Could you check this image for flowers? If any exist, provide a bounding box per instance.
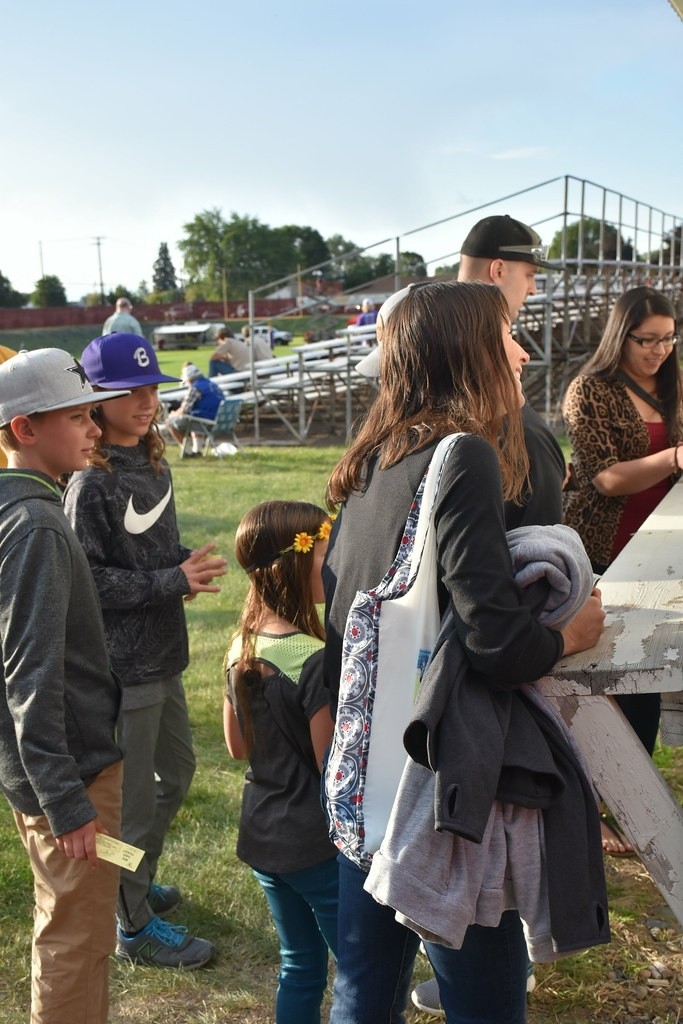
[291,514,339,554]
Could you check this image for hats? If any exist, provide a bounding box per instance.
[0,347,133,428]
[461,215,568,271]
[179,365,202,385]
[80,330,183,389]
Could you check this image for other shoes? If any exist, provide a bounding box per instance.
[411,973,535,1016]
[193,451,204,458]
[176,451,196,458]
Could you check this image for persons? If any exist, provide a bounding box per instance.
[456,216,559,320]
[62,331,229,969]
[101,297,146,340]
[560,286,683,859]
[0,347,128,1024]
[166,365,225,457]
[218,501,410,1024]
[239,327,272,379]
[209,329,253,394]
[312,279,606,1022]
[353,284,566,531]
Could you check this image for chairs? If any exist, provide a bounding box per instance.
[179,398,242,460]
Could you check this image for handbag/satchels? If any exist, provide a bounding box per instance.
[322,433,478,876]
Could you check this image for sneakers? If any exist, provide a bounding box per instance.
[115,882,181,922]
[115,915,214,970]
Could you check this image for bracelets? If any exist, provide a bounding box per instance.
[674,441,683,471]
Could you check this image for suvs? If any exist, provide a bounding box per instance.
[253,326,292,345]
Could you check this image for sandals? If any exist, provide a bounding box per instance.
[597,812,636,857]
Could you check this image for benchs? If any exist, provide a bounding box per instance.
[151,277,683,431]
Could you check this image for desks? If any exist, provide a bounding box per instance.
[527,479,683,933]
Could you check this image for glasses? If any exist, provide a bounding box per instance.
[498,244,549,262]
[627,331,680,348]
